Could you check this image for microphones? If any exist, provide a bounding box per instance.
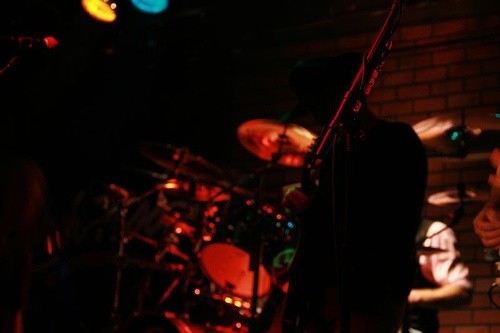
[0,35,60,51]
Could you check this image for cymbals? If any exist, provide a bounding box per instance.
[144,141,232,182]
[426,186,491,207]
[154,176,231,203]
[410,115,500,144]
[235,118,322,168]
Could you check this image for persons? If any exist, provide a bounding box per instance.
[277,55,426,332]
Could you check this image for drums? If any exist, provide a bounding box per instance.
[183,240,270,321]
